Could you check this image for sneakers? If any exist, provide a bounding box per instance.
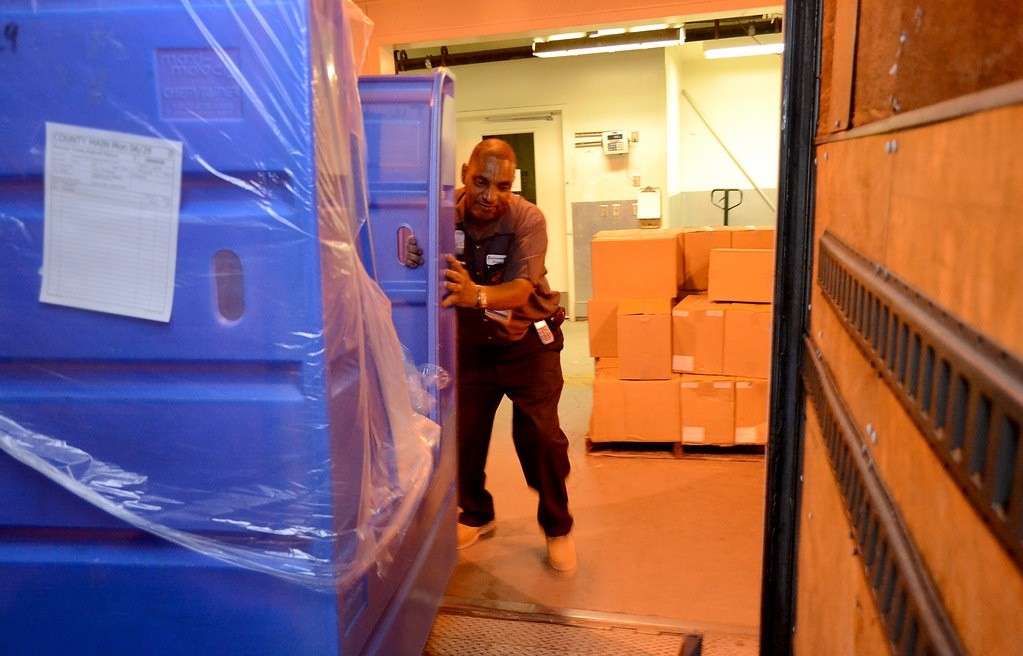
[547,532,577,571]
[456,517,497,550]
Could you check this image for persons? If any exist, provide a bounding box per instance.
[405,139,577,573]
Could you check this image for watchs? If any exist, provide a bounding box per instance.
[476,284,489,311]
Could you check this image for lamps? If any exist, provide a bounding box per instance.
[702,34,784,61]
[530,23,686,57]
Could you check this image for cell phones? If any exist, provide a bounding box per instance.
[534,320,555,344]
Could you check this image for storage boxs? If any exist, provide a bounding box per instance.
[588,234,679,299]
[589,299,617,357]
[735,377,770,443]
[614,298,673,380]
[707,248,775,304]
[626,373,681,442]
[685,225,732,291]
[681,373,735,444]
[594,369,626,443]
[723,302,773,379]
[595,356,618,369]
[591,230,685,290]
[670,293,732,375]
[733,225,775,249]
